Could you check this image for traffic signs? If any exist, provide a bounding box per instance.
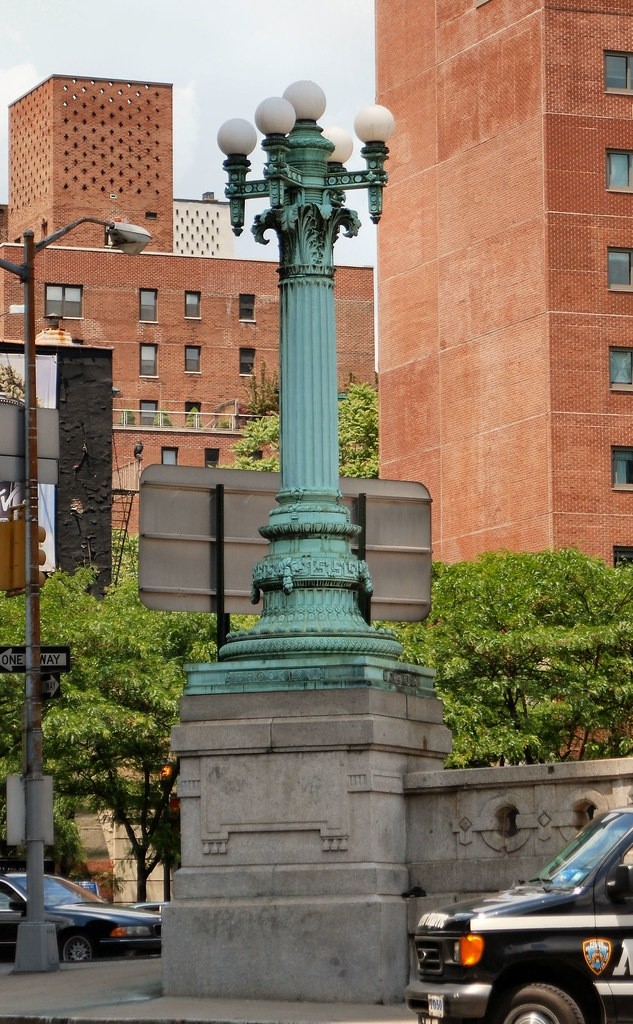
[40,673,62,700]
[0,645,71,674]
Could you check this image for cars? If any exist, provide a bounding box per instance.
[0,872,161,961]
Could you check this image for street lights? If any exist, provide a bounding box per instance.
[23,519,47,590]
[0,215,154,976]
[186,77,442,694]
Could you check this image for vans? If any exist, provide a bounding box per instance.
[403,804,633,1024]
